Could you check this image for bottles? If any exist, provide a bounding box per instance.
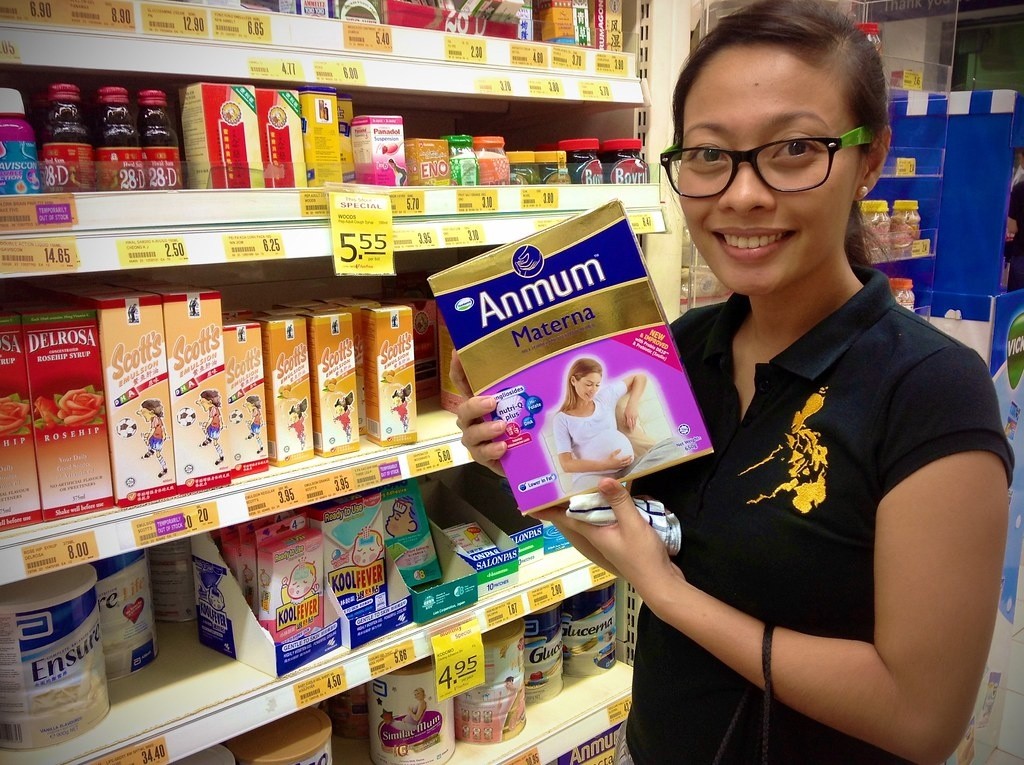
[137,90,183,192]
[0,82,95,195]
[859,200,891,255]
[95,87,145,194]
[891,200,920,255]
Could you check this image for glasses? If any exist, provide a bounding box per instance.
[660,126,872,198]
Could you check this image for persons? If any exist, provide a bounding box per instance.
[497,676,519,737]
[553,354,645,496]
[389,159,407,186]
[1008,148,1024,291]
[449,1,1017,764]
[1004,160,1024,271]
[390,687,427,732]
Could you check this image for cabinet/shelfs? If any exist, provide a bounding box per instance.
[0,0,691,765]
[681,0,958,324]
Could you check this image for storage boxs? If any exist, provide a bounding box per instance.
[0,276,475,530]
[191,467,572,680]
[240,0,623,49]
[427,201,715,517]
[178,81,407,188]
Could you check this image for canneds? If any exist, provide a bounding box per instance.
[170,579,618,765]
[443,134,652,184]
[0,537,197,752]
[861,200,921,313]
